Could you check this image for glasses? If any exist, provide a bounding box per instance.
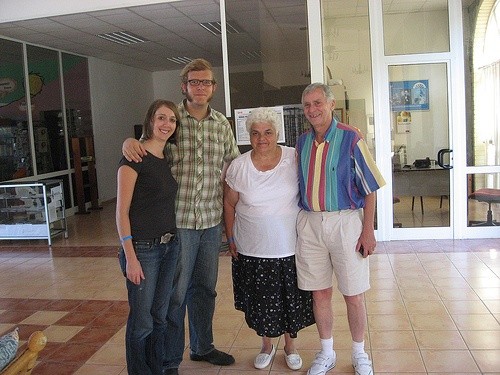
[187,79,214,86]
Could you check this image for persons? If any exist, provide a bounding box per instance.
[122,59,241,375]
[294,82,386,375]
[224,108,316,370]
[115,100,181,375]
[402,90,412,104]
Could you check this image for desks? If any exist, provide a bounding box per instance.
[393,165,449,215]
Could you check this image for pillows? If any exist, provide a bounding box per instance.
[0,327,19,371]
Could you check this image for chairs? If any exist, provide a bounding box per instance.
[0,330,47,375]
[468,188,500,226]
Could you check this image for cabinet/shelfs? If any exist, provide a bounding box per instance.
[0,179,69,246]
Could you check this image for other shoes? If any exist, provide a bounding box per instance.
[284,346,302,370]
[254,344,275,369]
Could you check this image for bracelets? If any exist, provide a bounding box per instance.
[228,238,233,243]
[121,235,132,241]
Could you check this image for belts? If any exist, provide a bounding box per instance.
[133,232,175,244]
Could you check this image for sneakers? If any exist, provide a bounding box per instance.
[190,349,235,366]
[351,353,373,375]
[306,350,336,375]
